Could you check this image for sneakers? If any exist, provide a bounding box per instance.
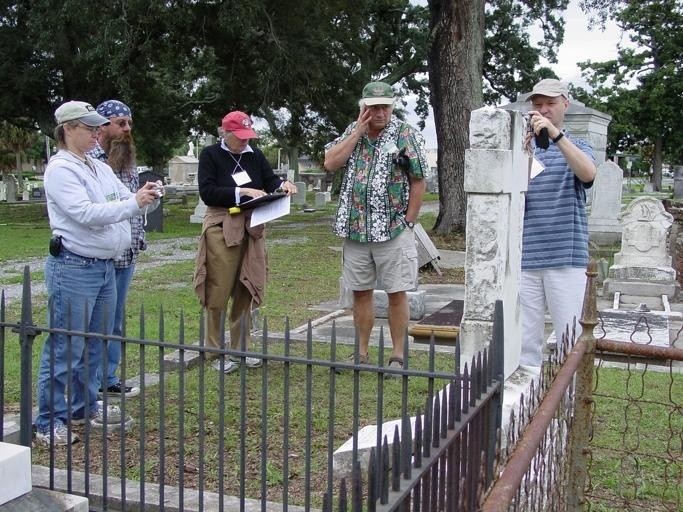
[98,383,139,397]
[384,357,403,379]
[35,423,80,446]
[229,355,263,368]
[334,352,368,374]
[70,399,103,426]
[205,359,237,374]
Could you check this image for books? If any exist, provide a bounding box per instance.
[236,191,291,229]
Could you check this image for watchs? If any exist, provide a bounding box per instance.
[405,220,415,228]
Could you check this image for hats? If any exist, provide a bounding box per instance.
[53,100,110,127]
[222,111,257,139]
[525,78,568,101]
[362,82,393,106]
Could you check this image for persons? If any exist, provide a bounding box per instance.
[517,75,599,422]
[33,98,165,447]
[195,110,301,373]
[85,99,145,398]
[324,79,423,383]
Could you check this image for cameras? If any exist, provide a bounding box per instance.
[522,113,534,132]
[151,183,164,199]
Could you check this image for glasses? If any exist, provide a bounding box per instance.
[77,127,99,132]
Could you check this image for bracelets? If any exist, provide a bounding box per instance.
[550,128,567,144]
